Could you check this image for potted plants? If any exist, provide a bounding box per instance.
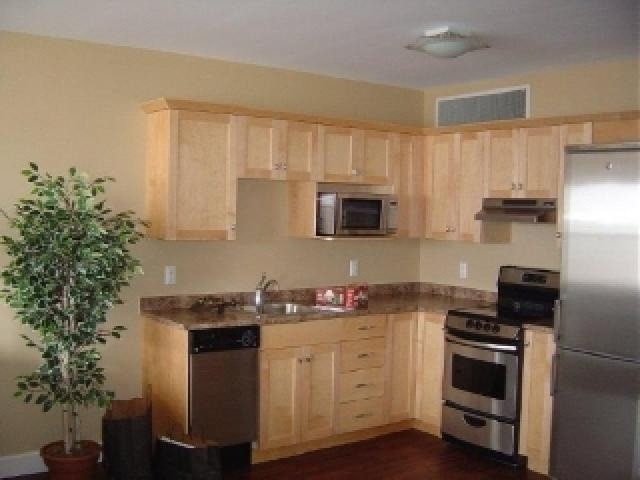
[0,160,148,480]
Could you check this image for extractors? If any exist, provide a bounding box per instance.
[473,197,557,224]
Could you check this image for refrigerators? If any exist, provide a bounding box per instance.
[547,140,640,480]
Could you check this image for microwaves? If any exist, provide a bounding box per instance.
[320,192,400,235]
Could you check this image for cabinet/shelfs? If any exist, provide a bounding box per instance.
[488,117,560,199]
[237,106,317,180]
[338,313,390,445]
[390,309,419,433]
[426,124,512,243]
[317,114,399,185]
[522,323,555,476]
[251,319,338,464]
[142,98,237,241]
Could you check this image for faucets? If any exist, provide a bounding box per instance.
[255,276,282,307]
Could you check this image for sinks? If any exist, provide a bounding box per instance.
[244,302,327,315]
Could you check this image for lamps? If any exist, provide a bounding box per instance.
[405,32,491,58]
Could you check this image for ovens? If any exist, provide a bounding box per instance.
[496,266,560,328]
[442,328,519,425]
